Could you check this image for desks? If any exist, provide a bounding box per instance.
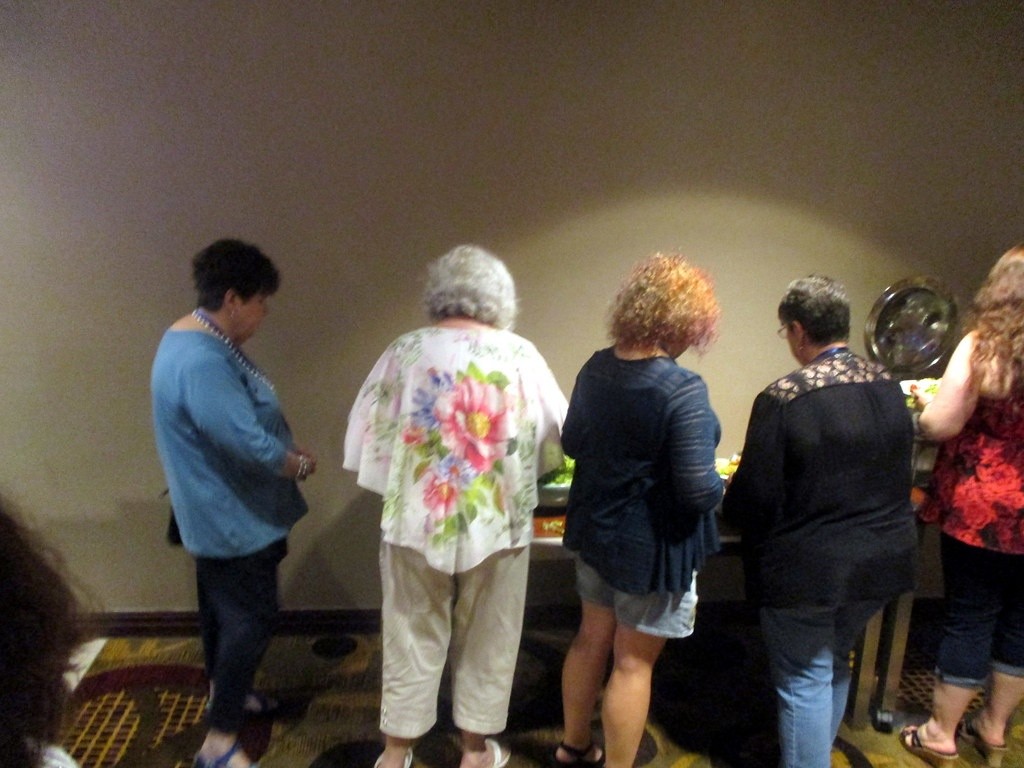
[528,482,942,732]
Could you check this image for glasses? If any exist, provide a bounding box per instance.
[778,319,804,339]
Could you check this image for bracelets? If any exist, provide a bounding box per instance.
[296,454,307,479]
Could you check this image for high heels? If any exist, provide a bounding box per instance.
[957,717,1009,768]
[899,723,959,768]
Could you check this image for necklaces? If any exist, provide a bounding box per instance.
[190,307,278,397]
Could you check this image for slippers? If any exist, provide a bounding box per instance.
[374,745,413,768]
[459,738,512,768]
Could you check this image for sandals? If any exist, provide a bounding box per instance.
[206,692,280,712]
[193,741,257,768]
[554,739,606,768]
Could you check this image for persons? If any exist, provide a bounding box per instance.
[725,275,914,768]
[149,237,318,767]
[0,498,106,768]
[900,246,1024,766]
[559,254,725,768]
[352,244,561,768]
